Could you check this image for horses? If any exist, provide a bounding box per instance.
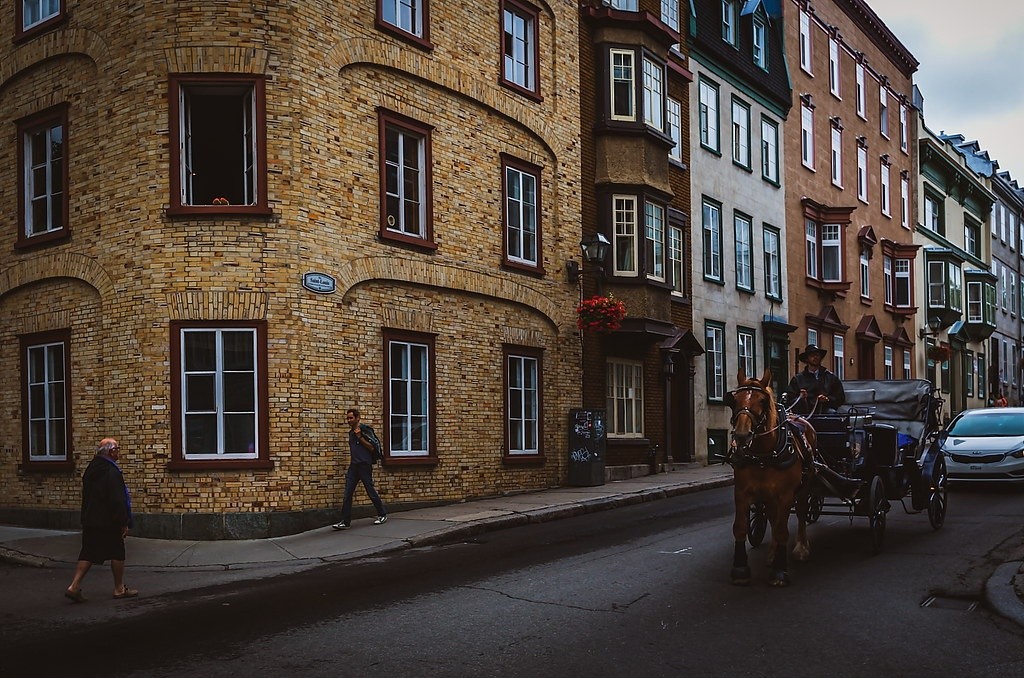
[730,367,817,587]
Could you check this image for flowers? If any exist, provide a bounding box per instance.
[928,346,954,363]
[577,292,626,338]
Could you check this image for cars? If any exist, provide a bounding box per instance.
[940,407,1024,486]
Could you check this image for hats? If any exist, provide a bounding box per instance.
[799,344,828,363]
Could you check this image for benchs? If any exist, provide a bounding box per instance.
[825,379,932,461]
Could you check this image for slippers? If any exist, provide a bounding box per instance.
[65,589,88,603]
[113,584,139,598]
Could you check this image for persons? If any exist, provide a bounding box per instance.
[988,392,1007,407]
[64,438,138,601]
[333,409,387,529]
[789,344,846,415]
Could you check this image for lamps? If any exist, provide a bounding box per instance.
[661,354,674,381]
[566,233,610,283]
[920,315,942,339]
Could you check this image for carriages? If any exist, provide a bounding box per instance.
[727,378,948,583]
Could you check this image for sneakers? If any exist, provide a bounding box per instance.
[374,516,388,525]
[332,520,354,529]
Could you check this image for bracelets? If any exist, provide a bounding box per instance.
[358,434,363,441]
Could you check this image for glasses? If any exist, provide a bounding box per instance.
[112,447,120,452]
